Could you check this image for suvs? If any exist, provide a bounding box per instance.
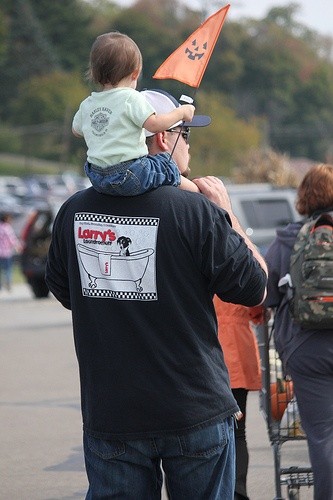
[229,184,306,256]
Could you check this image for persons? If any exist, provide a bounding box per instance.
[44,88,269,500]
[0,212,23,291]
[71,32,202,194]
[210,293,263,500]
[264,165,333,500]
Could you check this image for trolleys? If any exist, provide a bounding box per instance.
[255,307,314,500]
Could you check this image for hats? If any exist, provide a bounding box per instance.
[137,87,212,137]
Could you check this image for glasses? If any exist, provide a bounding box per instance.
[168,126,191,143]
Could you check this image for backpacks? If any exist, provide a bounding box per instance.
[278,212,333,331]
